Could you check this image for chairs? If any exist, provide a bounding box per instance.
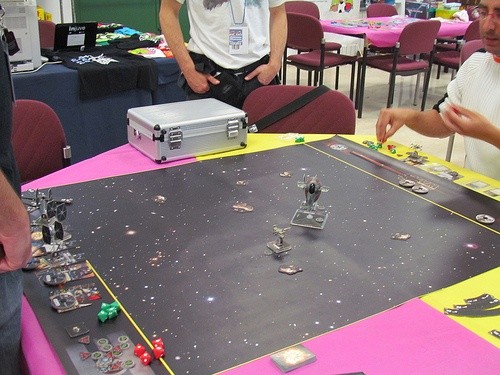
[243,0,500,134]
[12,99,71,186]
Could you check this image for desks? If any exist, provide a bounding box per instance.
[20,133,500,375]
[320,15,473,118]
[11,24,188,165]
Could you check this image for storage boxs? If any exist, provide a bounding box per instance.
[127,98,248,164]
[435,8,458,18]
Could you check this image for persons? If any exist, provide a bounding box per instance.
[376,0,500,181]
[158,0,288,109]
[0,37,32,375]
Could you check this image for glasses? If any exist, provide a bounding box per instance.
[468,6,500,20]
